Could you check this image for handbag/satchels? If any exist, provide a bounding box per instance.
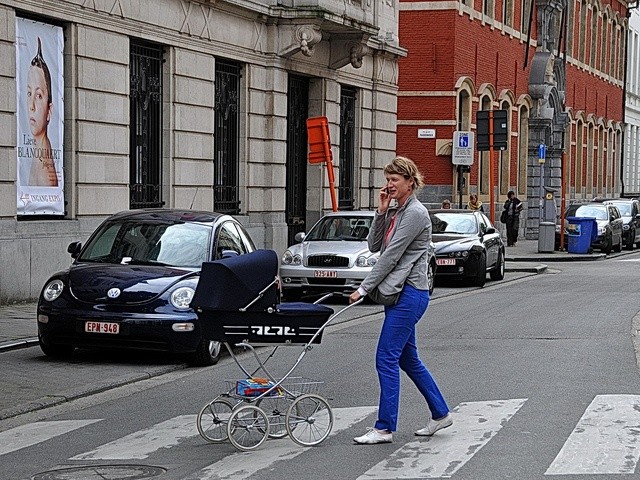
[368,287,400,306]
[500,208,509,223]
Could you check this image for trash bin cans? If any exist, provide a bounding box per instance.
[538,222,556,253]
[566,217,598,253]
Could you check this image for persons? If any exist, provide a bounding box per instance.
[464,194,484,214]
[27,36,58,185]
[349,157,453,445]
[441,200,451,210]
[504,191,524,248]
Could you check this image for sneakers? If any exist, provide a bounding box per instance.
[353,426,393,444]
[414,414,453,436]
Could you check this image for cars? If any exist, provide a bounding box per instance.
[280,209,437,304]
[592,196,640,251]
[423,209,507,289]
[553,202,623,253]
[36,208,257,367]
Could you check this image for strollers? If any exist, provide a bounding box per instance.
[185,249,366,451]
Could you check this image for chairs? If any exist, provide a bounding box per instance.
[350,224,370,239]
[333,226,353,239]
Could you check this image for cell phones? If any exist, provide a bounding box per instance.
[385,187,389,195]
